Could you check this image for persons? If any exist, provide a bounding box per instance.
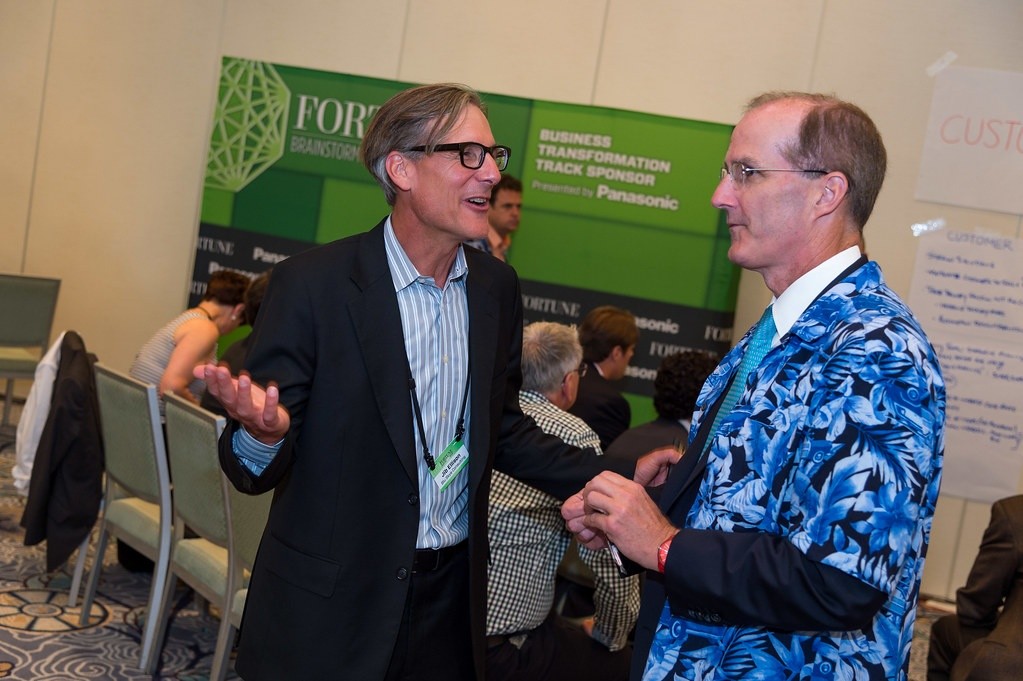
[486,306,720,681]
[561,91,946,681]
[193,83,683,681]
[128,269,272,425]
[927,490,1022,681]
[462,173,524,265]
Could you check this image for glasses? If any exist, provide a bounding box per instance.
[408,141,511,172]
[720,161,830,189]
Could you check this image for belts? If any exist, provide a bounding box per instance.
[410,540,468,580]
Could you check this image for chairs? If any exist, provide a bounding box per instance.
[57,333,176,675]
[141,389,253,681]
[208,427,276,681]
[0,274,60,427]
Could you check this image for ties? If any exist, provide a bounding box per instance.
[699,304,778,458]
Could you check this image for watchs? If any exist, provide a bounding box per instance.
[658,528,682,574]
